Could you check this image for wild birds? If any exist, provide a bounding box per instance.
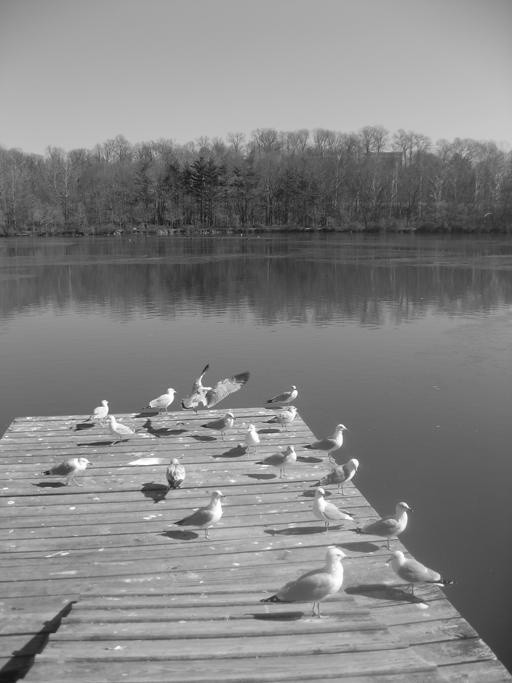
[309,458,359,496]
[301,423,349,465]
[86,387,177,447]
[167,458,185,489]
[312,487,360,534]
[180,364,298,479]
[386,551,456,597]
[41,457,93,487]
[348,502,413,551]
[259,547,351,619]
[173,490,226,541]
